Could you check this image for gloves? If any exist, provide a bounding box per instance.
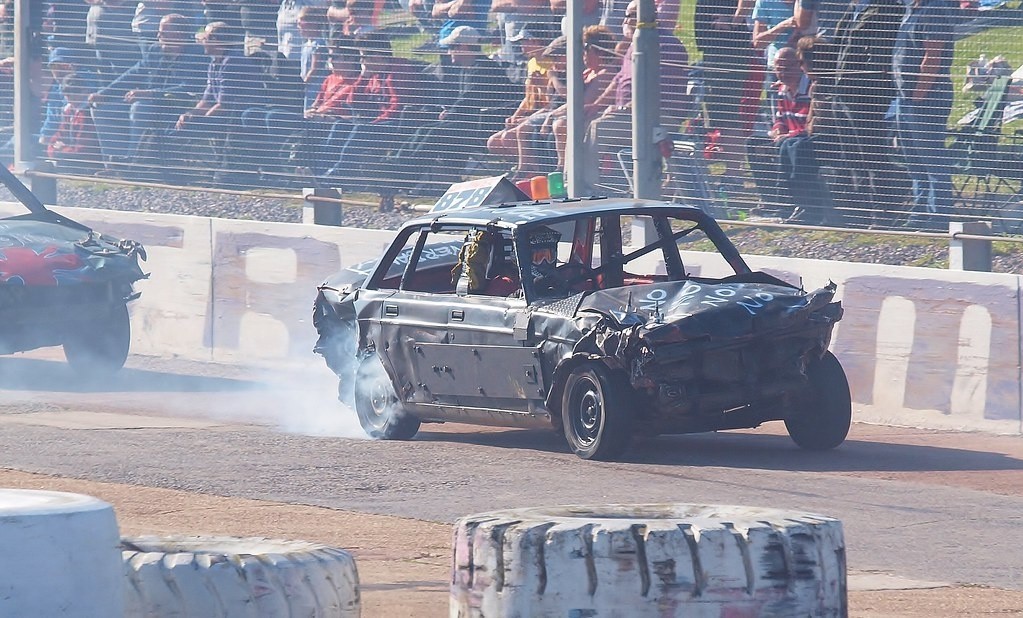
[533,278,546,295]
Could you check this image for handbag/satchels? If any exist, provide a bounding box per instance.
[963,60,1013,90]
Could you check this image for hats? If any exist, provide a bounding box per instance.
[440,26,479,46]
[47,46,73,67]
[510,28,534,41]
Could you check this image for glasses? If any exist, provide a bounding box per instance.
[447,45,461,51]
[531,248,558,265]
[63,88,82,94]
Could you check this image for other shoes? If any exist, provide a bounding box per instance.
[902,219,947,229]
[296,166,319,189]
[790,210,819,220]
[95,170,122,177]
[511,175,535,183]
[751,208,766,215]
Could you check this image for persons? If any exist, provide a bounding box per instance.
[486,225,604,297]
[1,0,953,232]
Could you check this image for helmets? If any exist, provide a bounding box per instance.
[510,226,562,278]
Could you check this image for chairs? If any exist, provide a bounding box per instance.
[947,76,1011,233]
[484,232,515,279]
[464,98,520,181]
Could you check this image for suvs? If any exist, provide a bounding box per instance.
[312,195,852,462]
[0,166,153,381]
